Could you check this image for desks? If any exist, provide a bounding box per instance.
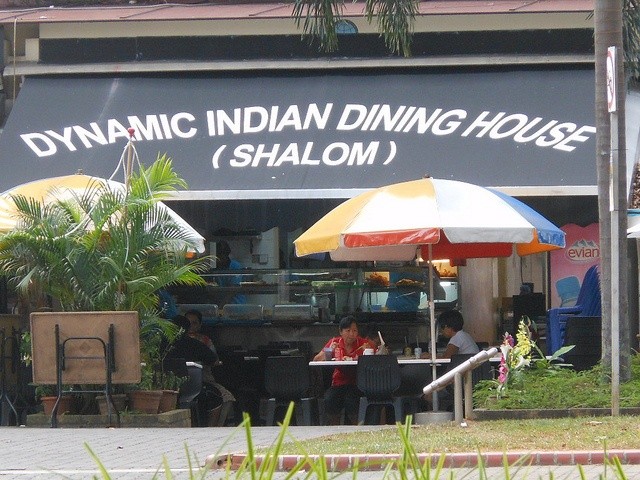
[30,310,141,426]
[1,313,34,424]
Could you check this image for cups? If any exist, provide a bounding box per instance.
[324,348,332,361]
[318,308,331,322]
[404,347,412,357]
[363,349,374,355]
[414,348,422,358]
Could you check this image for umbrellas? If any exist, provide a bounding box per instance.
[1,169,207,258]
[293,172,567,413]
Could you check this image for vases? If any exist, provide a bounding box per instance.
[94,393,128,413]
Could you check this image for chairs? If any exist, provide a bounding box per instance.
[356,356,401,426]
[152,356,204,426]
[546,263,601,357]
[442,353,492,418]
[259,341,312,351]
[264,356,311,425]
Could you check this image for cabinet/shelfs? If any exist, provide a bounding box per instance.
[174,267,431,327]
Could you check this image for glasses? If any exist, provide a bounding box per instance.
[441,324,448,329]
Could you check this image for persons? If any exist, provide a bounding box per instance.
[420,310,480,410]
[385,270,446,311]
[183,309,237,427]
[211,240,248,305]
[313,316,381,425]
[155,315,223,427]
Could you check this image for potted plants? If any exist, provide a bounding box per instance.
[32,383,78,417]
[127,369,162,415]
[159,370,177,415]
